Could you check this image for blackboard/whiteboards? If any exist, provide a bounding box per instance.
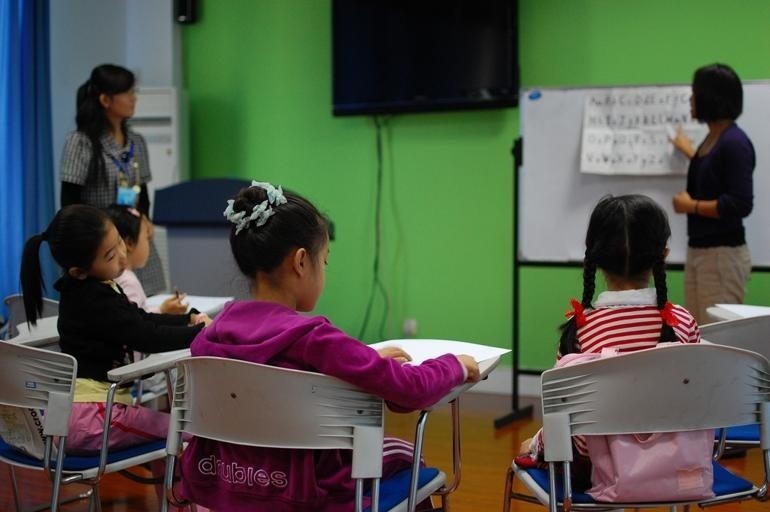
[519,78,770,273]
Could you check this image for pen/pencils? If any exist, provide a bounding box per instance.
[176,290,179,298]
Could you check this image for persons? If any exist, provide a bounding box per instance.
[180,181,480,512]
[666,63,756,327]
[19,206,214,511]
[59,64,167,297]
[519,194,702,481]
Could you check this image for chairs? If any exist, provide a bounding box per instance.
[503,303,769,512]
[0,294,238,512]
[161,337,501,512]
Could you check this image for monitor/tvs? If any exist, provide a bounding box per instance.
[331,0,520,117]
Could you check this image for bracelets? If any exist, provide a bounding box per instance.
[695,199,699,214]
[195,312,208,325]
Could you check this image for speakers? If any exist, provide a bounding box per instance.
[171,0,203,25]
